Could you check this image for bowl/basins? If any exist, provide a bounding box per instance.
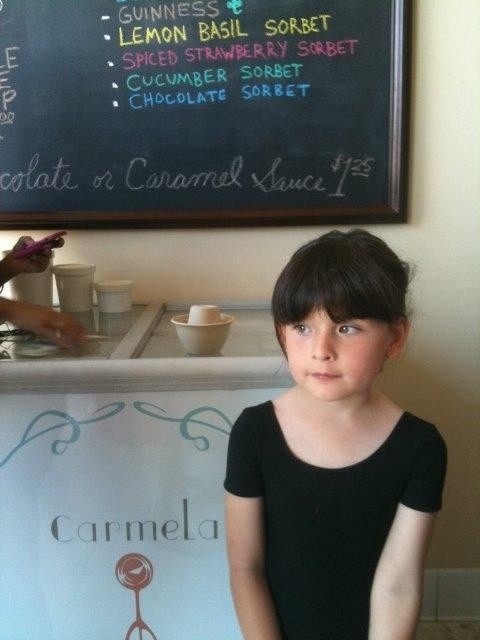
[169,313,235,355]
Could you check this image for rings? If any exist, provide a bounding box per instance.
[56,329,62,338]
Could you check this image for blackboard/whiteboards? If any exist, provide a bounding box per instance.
[0,0,413,231]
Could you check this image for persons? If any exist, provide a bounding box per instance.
[221,227,448,640]
[0,235,85,351]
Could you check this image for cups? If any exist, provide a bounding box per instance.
[51,262,97,314]
[94,279,135,313]
[3,249,55,311]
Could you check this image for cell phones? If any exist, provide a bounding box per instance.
[13,229,66,260]
